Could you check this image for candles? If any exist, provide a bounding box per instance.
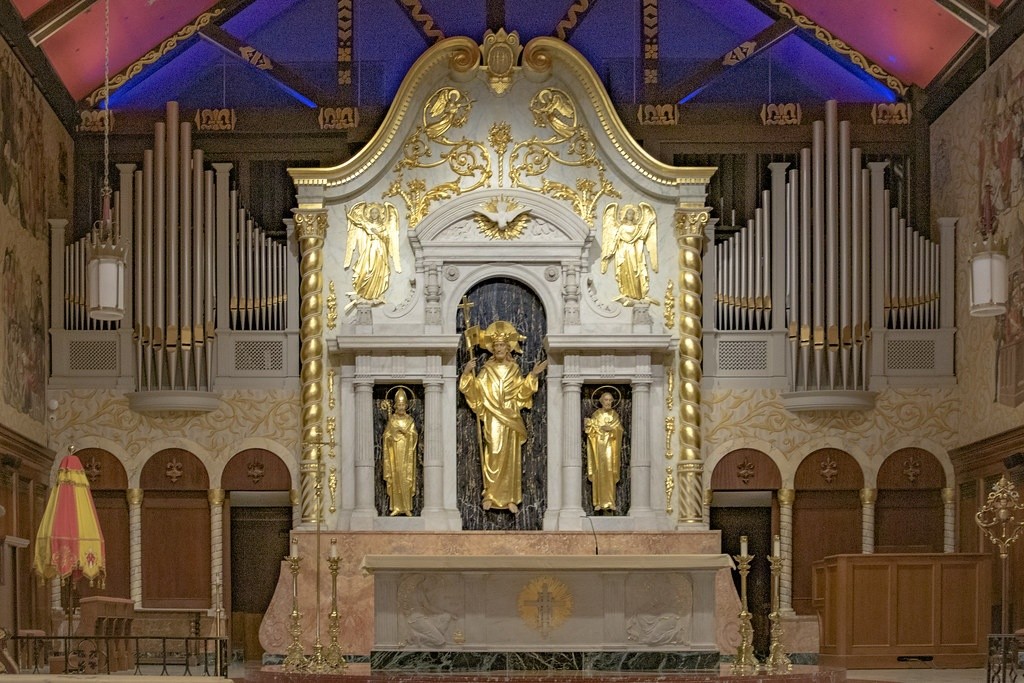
[773,535,780,556]
[330,537,338,558]
[292,536,298,557]
[216,572,219,584]
[739,536,748,558]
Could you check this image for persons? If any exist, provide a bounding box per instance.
[533,92,578,140]
[614,208,649,301]
[426,90,478,136]
[458,321,549,514]
[381,384,419,518]
[352,208,390,301]
[583,392,624,511]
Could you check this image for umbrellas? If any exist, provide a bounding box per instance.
[28,442,109,658]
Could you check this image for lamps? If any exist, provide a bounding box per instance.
[969,0,1009,318]
[85,0,125,319]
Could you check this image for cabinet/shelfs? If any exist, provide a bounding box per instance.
[812,553,994,670]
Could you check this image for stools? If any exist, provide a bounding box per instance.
[17,629,45,669]
